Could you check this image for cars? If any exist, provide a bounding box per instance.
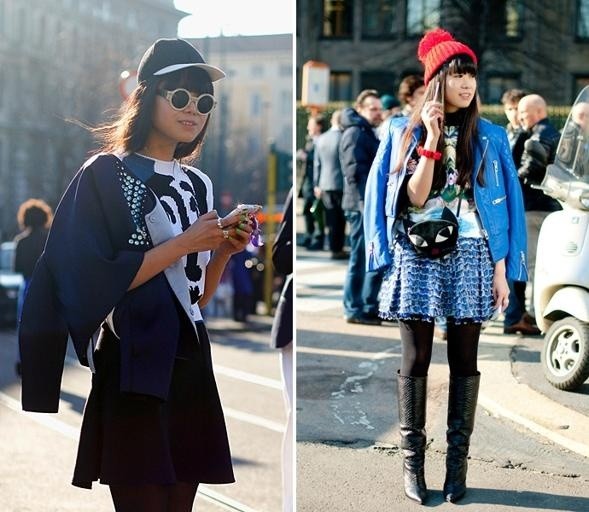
[245,201,286,314]
[0,240,27,328]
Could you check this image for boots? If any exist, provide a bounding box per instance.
[443,371,480,504]
[397,371,429,505]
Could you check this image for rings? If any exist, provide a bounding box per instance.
[216,218,222,229]
[222,231,229,239]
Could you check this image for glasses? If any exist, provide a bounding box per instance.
[157,84,218,116]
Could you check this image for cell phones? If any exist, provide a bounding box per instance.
[223,203,262,230]
[434,82,443,129]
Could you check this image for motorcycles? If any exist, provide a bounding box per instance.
[522,82,589,395]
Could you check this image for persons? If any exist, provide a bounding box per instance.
[361,29,530,504]
[14,200,54,379]
[228,241,255,323]
[17,37,261,512]
[296,74,560,336]
[558,102,588,182]
[270,181,294,509]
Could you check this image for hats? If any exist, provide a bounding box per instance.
[137,38,226,87]
[417,28,479,86]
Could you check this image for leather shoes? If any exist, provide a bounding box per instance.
[504,314,542,336]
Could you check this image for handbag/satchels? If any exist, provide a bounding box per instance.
[401,206,459,261]
